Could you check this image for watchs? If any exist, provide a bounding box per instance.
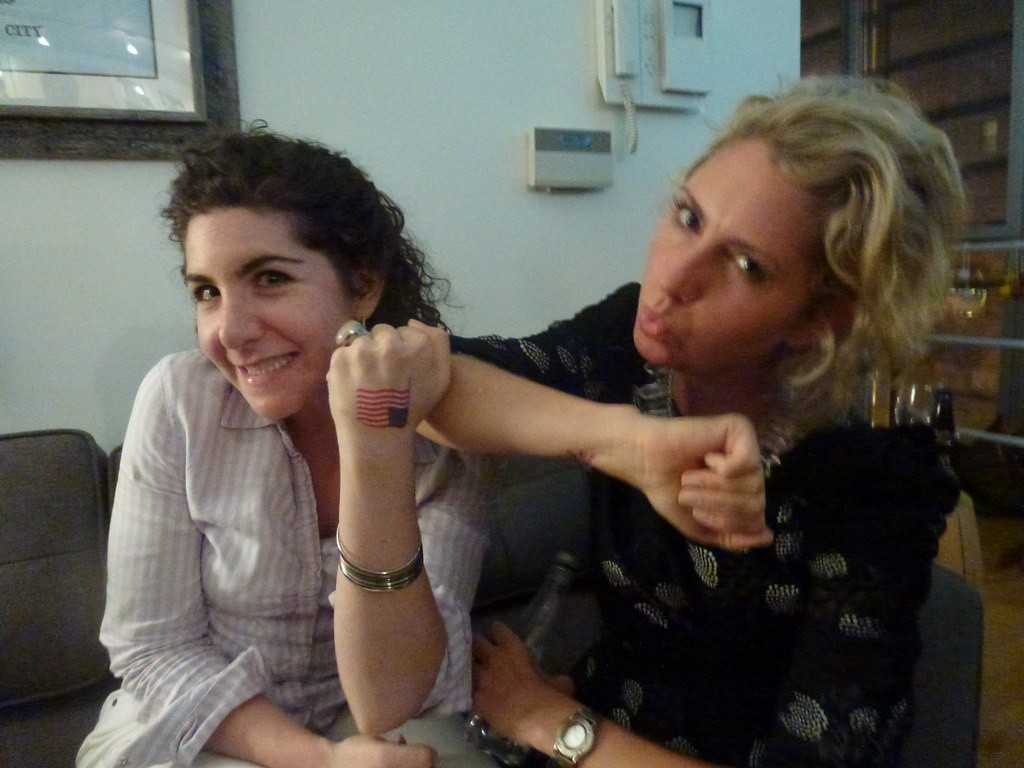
[550,708,606,768]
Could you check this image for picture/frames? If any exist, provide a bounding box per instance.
[0,0,241,162]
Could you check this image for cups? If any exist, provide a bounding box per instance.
[894,383,931,427]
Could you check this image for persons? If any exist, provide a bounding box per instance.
[72,123,506,768]
[409,73,965,768]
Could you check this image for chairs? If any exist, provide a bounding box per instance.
[0,427,124,768]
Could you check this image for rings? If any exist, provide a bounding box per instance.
[337,325,372,347]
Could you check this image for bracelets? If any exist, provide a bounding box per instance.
[335,520,425,593]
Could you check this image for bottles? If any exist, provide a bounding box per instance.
[931,389,967,491]
[465,552,579,763]
